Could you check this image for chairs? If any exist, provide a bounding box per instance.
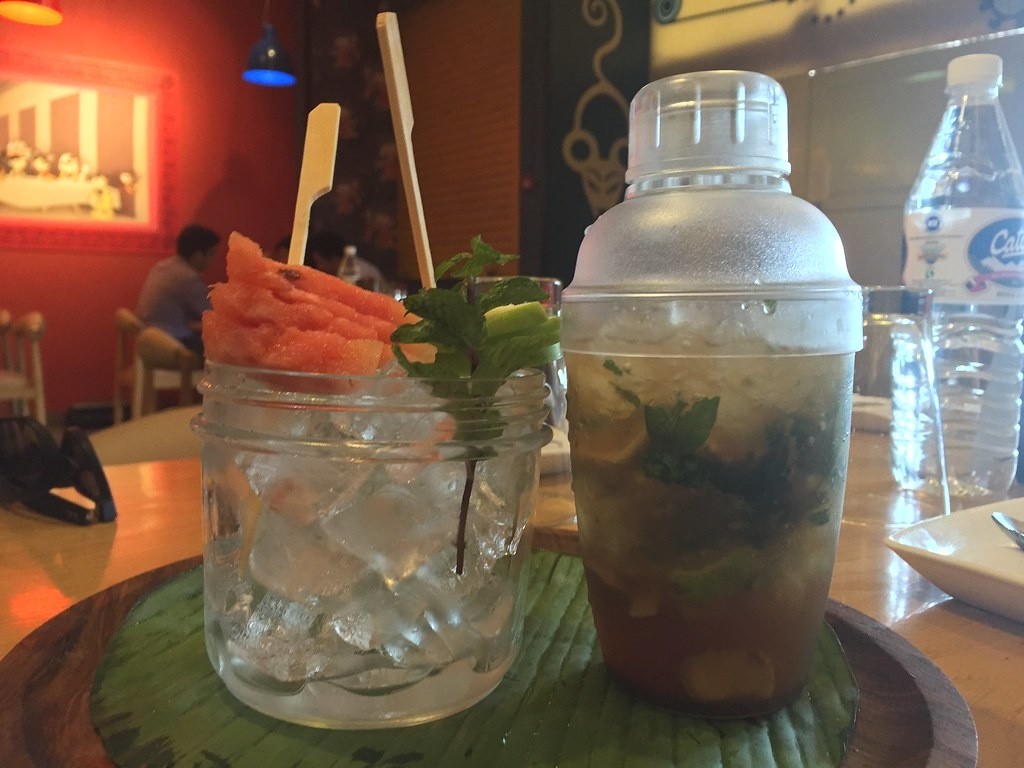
[112,306,200,426]
[0,307,49,427]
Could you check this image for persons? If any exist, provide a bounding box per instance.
[135,224,222,352]
[272,171,386,295]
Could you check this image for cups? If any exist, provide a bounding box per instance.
[191,360,553,730]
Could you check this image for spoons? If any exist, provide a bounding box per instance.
[990,511,1024,549]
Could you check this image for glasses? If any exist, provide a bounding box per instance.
[1,416,118,526]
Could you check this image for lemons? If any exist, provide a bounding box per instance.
[575,408,650,472]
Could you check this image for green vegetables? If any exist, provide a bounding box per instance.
[388,238,838,577]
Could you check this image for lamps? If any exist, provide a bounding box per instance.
[241,0,297,87]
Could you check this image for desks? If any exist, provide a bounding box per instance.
[0,426,1023,768]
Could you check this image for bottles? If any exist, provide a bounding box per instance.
[889,52,1023,498]
[558,70,863,721]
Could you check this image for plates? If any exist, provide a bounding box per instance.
[885,499,1023,627]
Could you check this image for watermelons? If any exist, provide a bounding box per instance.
[199,231,429,393]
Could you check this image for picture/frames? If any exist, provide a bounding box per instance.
[0,47,176,257]
